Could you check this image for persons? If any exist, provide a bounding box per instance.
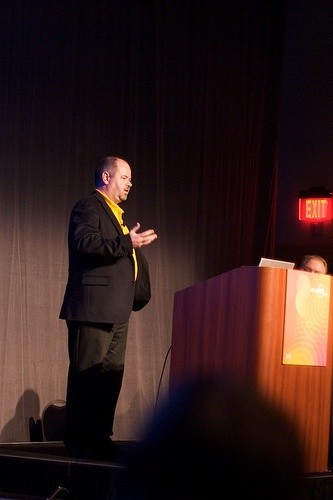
[300,255,327,275]
[59,158,157,460]
